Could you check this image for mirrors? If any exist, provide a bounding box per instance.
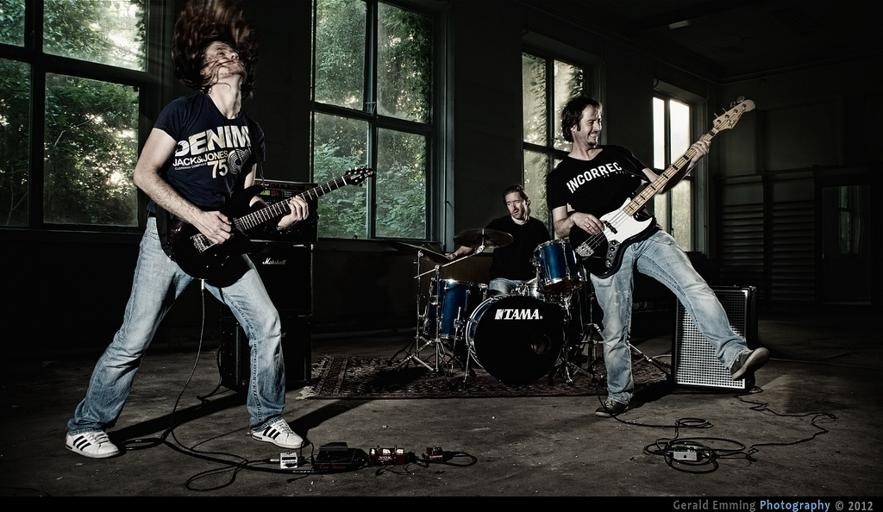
[815,175,878,314]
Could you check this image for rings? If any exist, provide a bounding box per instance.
[585,224,591,228]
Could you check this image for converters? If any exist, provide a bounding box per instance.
[314,447,360,471]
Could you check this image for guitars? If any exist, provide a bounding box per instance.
[569,96,757,279]
[169,166,377,280]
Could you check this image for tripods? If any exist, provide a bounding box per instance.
[549,287,672,385]
[389,250,485,383]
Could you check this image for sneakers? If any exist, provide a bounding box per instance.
[596,401,627,419]
[730,348,769,381]
[65,430,119,459]
[252,417,304,450]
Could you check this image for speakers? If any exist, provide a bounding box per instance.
[220,239,315,394]
[672,285,758,392]
[253,178,319,241]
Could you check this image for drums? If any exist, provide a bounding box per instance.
[522,281,571,320]
[423,278,482,341]
[531,241,587,294]
[466,294,568,389]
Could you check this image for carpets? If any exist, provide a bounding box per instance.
[296,350,671,402]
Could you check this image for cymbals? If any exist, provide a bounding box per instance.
[400,241,451,265]
[454,227,513,249]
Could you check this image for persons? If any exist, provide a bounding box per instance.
[481,185,553,296]
[549,97,772,419]
[65,1,317,460]
